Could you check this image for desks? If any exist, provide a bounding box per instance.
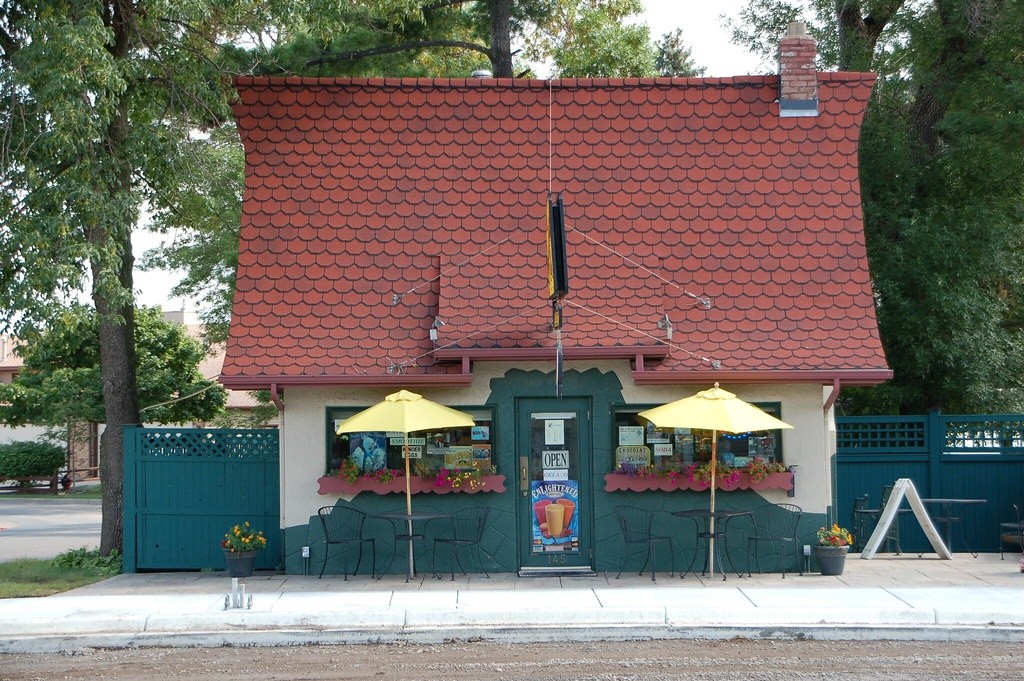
[855,508,913,550]
[671,509,754,581]
[920,498,988,558]
[371,511,449,580]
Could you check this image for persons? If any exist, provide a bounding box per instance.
[351,435,386,472]
[435,434,447,448]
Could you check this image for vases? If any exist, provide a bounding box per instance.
[225,551,257,577]
[815,546,850,575]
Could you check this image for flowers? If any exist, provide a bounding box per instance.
[221,522,267,553]
[819,523,853,548]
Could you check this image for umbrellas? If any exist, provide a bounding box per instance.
[637,381,793,583]
[337,389,476,579]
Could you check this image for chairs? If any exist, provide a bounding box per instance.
[614,505,674,581]
[852,485,904,556]
[318,505,375,579]
[745,503,804,578]
[998,504,1024,560]
[432,506,490,580]
